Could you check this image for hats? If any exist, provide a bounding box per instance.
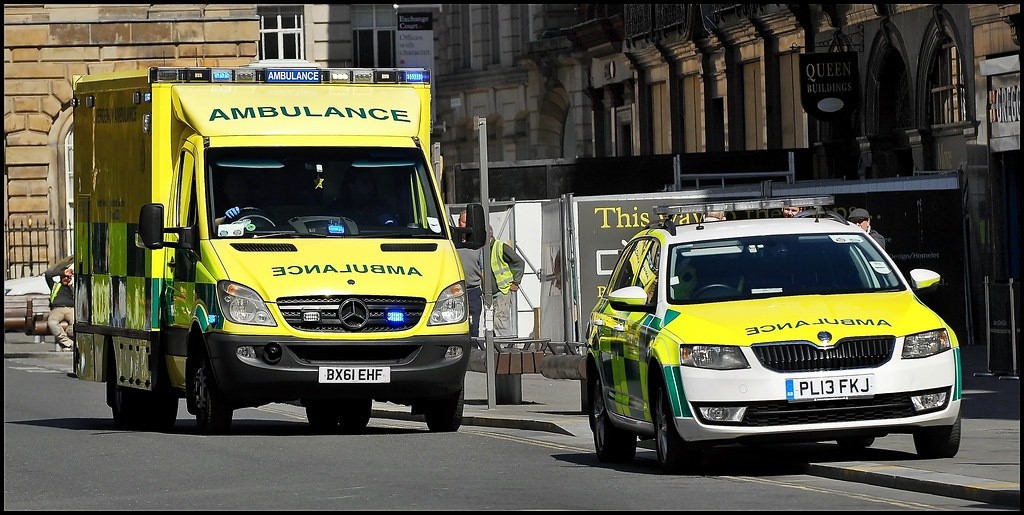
[848,208,872,219]
[490,225,493,237]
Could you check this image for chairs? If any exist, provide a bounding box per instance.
[320,172,397,226]
[781,252,839,290]
[682,257,744,296]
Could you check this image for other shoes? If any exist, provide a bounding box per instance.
[55,341,63,352]
[63,346,73,352]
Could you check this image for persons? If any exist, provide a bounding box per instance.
[702,211,727,222]
[215,174,277,224]
[452,210,483,337]
[45,268,75,353]
[848,209,887,250]
[781,206,802,219]
[321,171,402,227]
[478,225,525,347]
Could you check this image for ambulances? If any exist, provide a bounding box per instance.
[581,194,964,475]
[66,56,491,439]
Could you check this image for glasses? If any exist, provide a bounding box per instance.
[226,182,249,188]
[850,218,868,224]
[61,274,71,279]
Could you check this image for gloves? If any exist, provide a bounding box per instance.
[224,206,240,219]
